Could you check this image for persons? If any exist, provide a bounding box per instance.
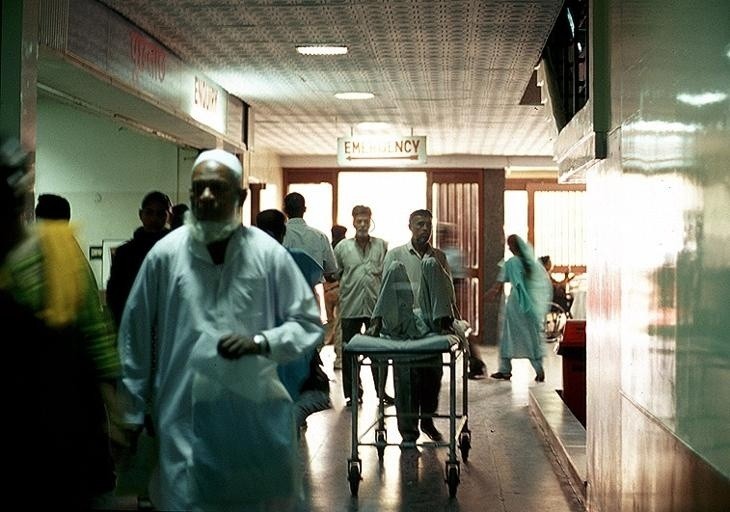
[34,192,71,225]
[282,192,340,283]
[255,209,332,427]
[538,255,577,315]
[483,234,553,382]
[0,122,124,511]
[364,257,487,379]
[334,204,396,406]
[315,224,348,369]
[367,208,465,447]
[435,221,466,322]
[115,148,322,512]
[105,190,172,338]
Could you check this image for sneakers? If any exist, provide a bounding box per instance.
[492,373,511,379]
[401,433,419,449]
[421,419,441,441]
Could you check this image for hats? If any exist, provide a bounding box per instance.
[192,149,242,177]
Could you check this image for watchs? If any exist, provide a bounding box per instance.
[253,333,268,356]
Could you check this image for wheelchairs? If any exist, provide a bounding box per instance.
[536,291,576,343]
